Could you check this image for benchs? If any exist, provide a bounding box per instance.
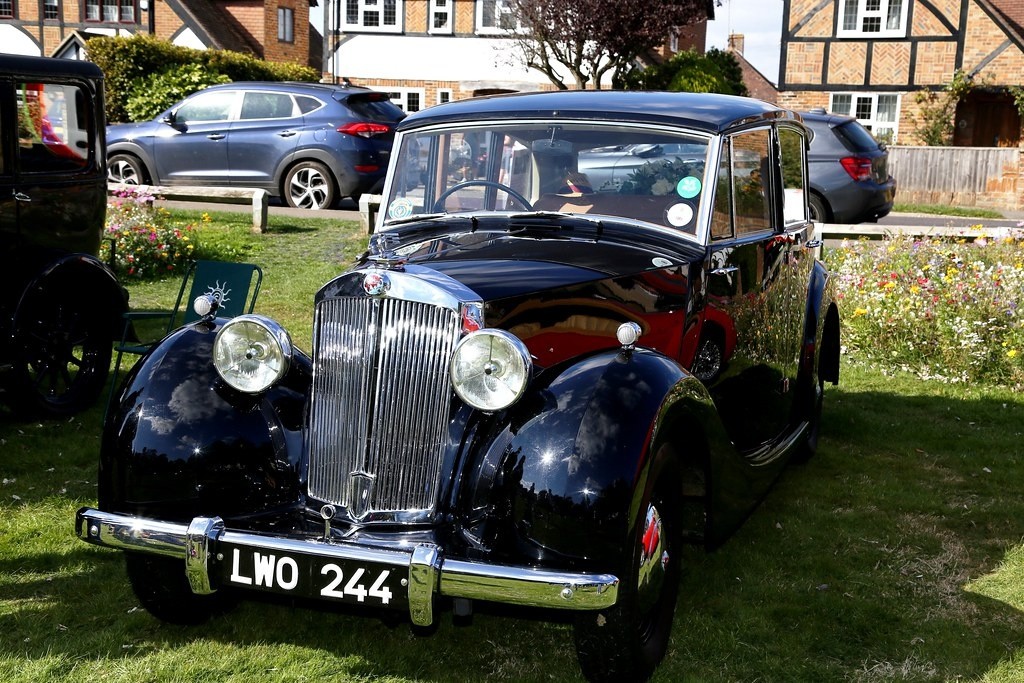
[533,193,717,235]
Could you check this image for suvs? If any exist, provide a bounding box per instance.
[74,91,840,683]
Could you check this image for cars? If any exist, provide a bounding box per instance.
[104,79,418,209]
[578,106,897,224]
[0,52,130,423]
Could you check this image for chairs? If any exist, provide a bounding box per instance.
[103,260,262,424]
